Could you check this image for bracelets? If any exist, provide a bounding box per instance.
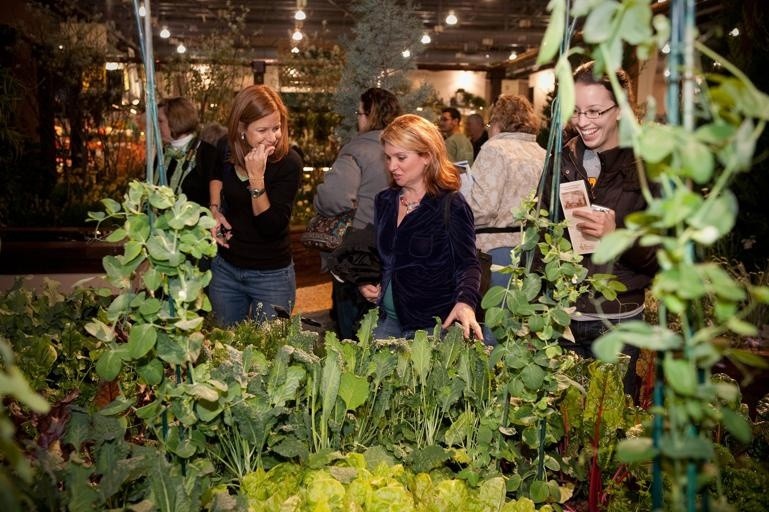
[208,202,220,210]
[246,175,266,181]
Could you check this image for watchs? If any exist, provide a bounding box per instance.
[245,185,268,199]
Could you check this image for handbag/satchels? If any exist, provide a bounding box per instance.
[298,153,364,252]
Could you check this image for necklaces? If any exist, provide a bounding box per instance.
[235,168,249,181]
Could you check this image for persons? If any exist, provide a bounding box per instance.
[354,111,486,383]
[464,112,489,163]
[517,57,679,411]
[463,91,556,354]
[200,121,229,149]
[312,86,402,345]
[440,106,476,176]
[192,83,306,332]
[143,94,216,320]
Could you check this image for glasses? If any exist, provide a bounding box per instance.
[568,105,618,120]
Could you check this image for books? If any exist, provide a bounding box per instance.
[557,178,604,256]
